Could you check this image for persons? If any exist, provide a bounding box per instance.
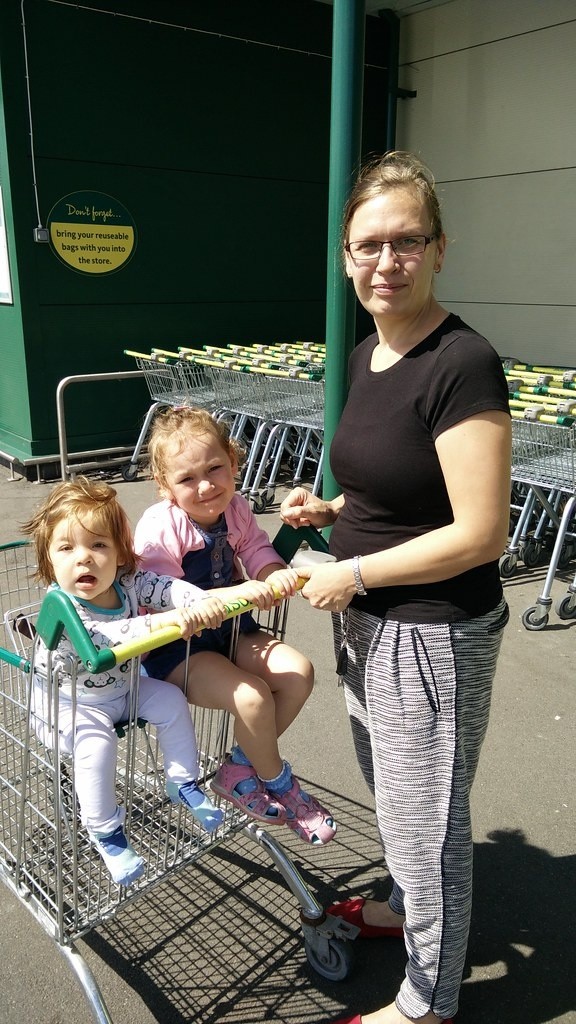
[20,476,223,885]
[281,162,514,1024]
[133,407,337,845]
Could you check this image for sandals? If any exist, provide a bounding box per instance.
[267,775,337,845]
[210,752,285,826]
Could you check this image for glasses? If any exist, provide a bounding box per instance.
[344,232,438,260]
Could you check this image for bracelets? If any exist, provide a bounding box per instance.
[353,555,367,596]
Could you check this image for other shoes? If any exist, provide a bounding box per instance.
[326,899,404,938]
[329,1013,451,1024]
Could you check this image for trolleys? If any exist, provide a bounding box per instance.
[1,541,362,1024]
[123,341,576,632]
